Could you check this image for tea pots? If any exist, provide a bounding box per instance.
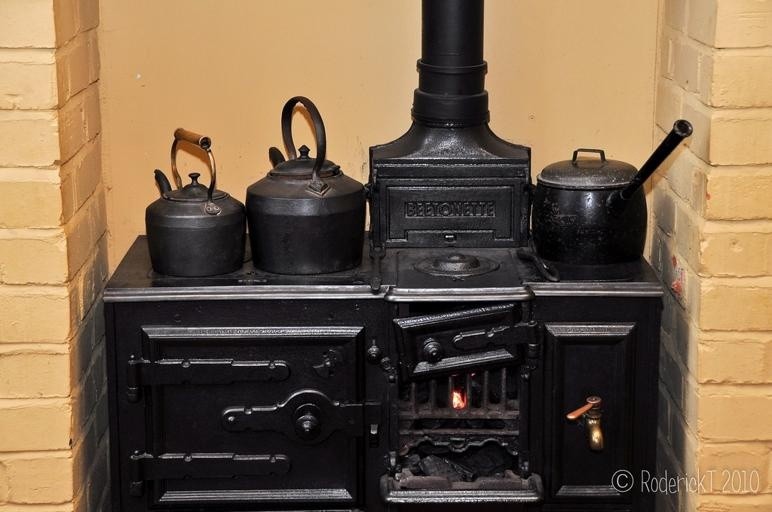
[145,127,246,275]
[246,97,366,276]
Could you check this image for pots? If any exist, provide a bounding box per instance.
[533,120,693,276]
